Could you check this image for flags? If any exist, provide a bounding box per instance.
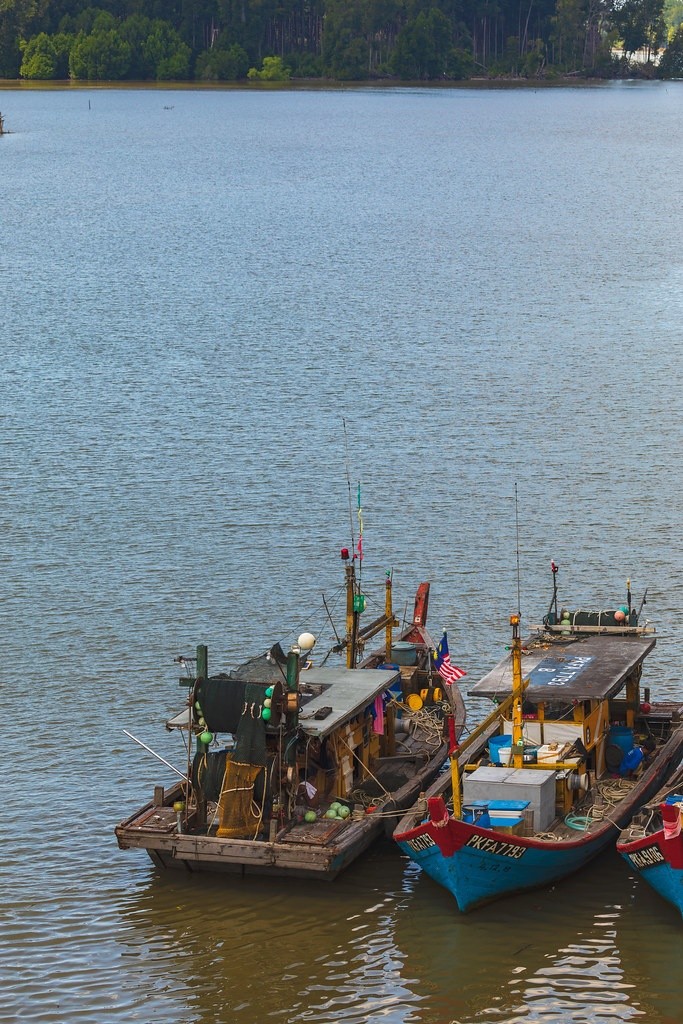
[432,631,469,685]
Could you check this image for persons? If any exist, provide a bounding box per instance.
[620,739,658,781]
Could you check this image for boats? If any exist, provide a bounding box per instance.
[390,480,683,917]
[113,417,467,888]
[617,757,682,919]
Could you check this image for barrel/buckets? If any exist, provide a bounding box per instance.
[421,688,442,702]
[462,805,490,829]
[406,694,423,709]
[498,748,511,764]
[391,641,417,666]
[606,727,634,774]
[488,734,512,764]
